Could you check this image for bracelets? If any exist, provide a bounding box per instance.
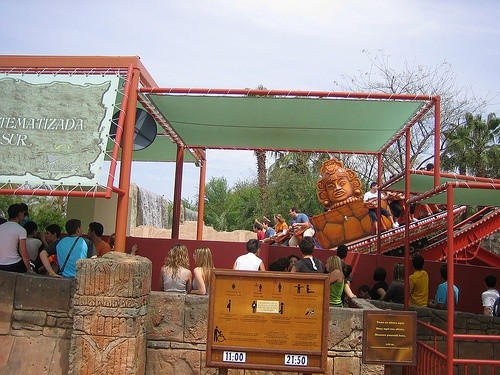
[48,271,55,276]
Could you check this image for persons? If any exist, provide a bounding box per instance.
[108,233,115,250]
[191,247,215,295]
[357,284,370,300]
[317,157,362,211]
[40,219,98,278]
[158,243,193,294]
[371,267,389,301]
[380,261,405,303]
[410,254,429,309]
[0,202,61,277]
[363,163,447,235]
[481,274,500,316]
[253,208,315,248]
[233,239,266,272]
[290,236,325,274]
[86,221,111,258]
[269,255,301,272]
[336,244,356,299]
[325,256,344,308]
[427,264,459,311]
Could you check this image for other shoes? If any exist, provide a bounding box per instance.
[410,217,419,222]
[392,223,398,228]
